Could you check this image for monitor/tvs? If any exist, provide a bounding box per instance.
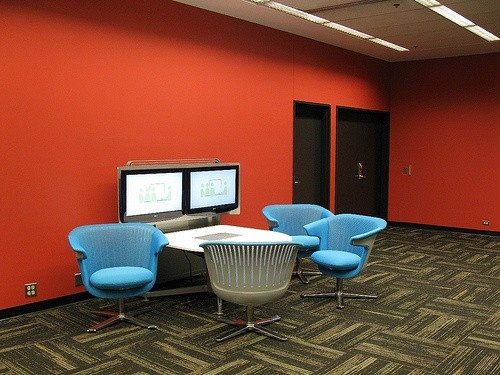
[120,167,186,224]
[186,165,239,216]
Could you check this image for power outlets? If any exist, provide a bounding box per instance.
[74,274,83,286]
[482,220,490,226]
[23,281,37,297]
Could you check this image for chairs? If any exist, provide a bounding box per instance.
[261,201,331,286]
[303,212,389,308]
[68,223,168,334]
[200,240,300,341]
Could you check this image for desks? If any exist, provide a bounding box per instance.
[165,224,290,321]
